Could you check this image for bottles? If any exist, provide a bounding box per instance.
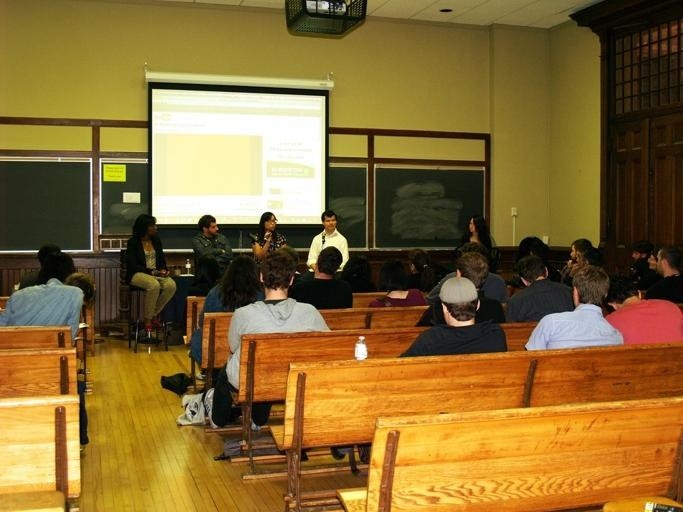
[354,336,367,359]
[185,259,191,274]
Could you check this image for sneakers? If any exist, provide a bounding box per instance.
[203,387,219,430]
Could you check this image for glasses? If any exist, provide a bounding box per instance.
[266,218,278,224]
[322,235,325,244]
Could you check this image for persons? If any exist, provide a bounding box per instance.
[330,277,507,463]
[191,215,233,278]
[504,236,683,350]
[388,215,506,358]
[191,255,265,380]
[0,245,96,444]
[255,212,288,264]
[306,210,349,273]
[342,255,376,292]
[204,249,329,430]
[289,246,353,309]
[127,214,177,332]
[280,245,301,282]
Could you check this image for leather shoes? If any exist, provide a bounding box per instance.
[142,319,152,332]
[152,319,161,331]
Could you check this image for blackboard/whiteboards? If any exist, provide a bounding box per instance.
[0,155,94,254]
[375,163,485,250]
[101,158,369,252]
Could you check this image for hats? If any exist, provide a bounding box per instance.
[37,244,62,262]
[631,240,654,253]
[438,277,478,304]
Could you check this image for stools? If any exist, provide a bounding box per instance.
[128,284,168,355]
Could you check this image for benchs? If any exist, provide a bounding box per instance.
[200,306,431,434]
[1,325,72,347]
[1,347,77,397]
[183,289,427,394]
[260,341,683,512]
[0,396,83,512]
[0,491,65,512]
[334,395,683,512]
[231,322,539,481]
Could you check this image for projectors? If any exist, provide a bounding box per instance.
[306,0,347,15]
